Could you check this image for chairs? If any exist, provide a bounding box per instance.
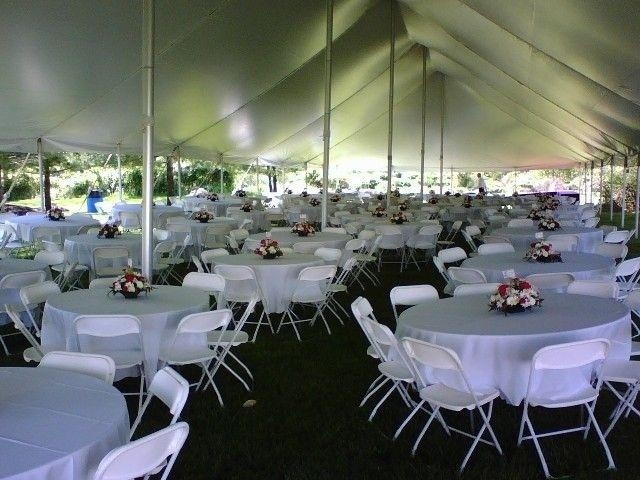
[36,351,116,386]
[566,280,619,298]
[393,337,502,472]
[342,189,640,292]
[93,422,189,480]
[454,282,502,297]
[584,358,640,440]
[0,188,381,413]
[128,366,190,480]
[389,285,440,321]
[351,296,414,421]
[398,293,631,338]
[626,288,640,357]
[517,338,616,478]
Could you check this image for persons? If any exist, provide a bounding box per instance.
[477,172,487,193]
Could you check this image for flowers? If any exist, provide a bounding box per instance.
[488,277,542,316]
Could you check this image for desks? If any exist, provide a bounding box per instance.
[0,366,126,480]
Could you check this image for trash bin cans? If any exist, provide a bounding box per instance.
[86,190,103,213]
